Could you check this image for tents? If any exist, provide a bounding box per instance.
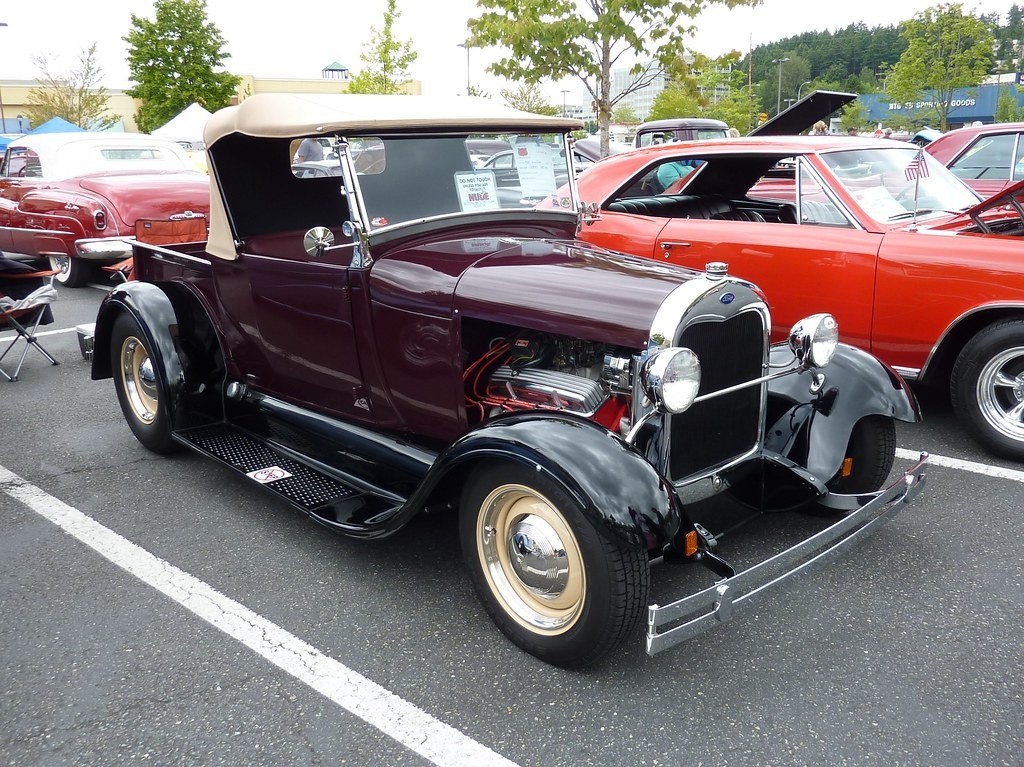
[0,101,214,152]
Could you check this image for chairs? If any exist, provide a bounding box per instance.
[0,252,63,383]
[101,206,208,286]
[778,200,836,222]
[710,210,767,223]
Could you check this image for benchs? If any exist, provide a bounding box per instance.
[243,216,358,262]
[607,195,736,220]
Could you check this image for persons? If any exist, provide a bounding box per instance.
[657,138,696,191]
[846,127,894,140]
[812,122,832,135]
[728,128,739,139]
[298,138,323,174]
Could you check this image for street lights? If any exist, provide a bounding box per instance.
[560,91,571,118]
[784,99,794,107]
[772,58,790,114]
[456,44,470,96]
[797,82,810,100]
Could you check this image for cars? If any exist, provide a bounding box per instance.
[0,132,211,289]
[352,116,734,208]
[750,120,1024,214]
[76,96,930,673]
[530,89,1024,466]
[288,160,341,178]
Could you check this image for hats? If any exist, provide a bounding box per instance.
[847,127,858,132]
[884,128,892,134]
[667,138,680,143]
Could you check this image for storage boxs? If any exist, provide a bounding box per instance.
[75,322,95,360]
